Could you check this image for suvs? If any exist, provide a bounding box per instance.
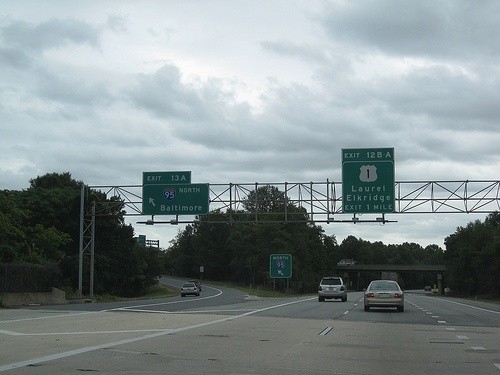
[318,276,347,302]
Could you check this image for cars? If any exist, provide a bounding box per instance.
[181,282,200,297]
[363,280,404,312]
[188,280,201,291]
[424,285,431,291]
